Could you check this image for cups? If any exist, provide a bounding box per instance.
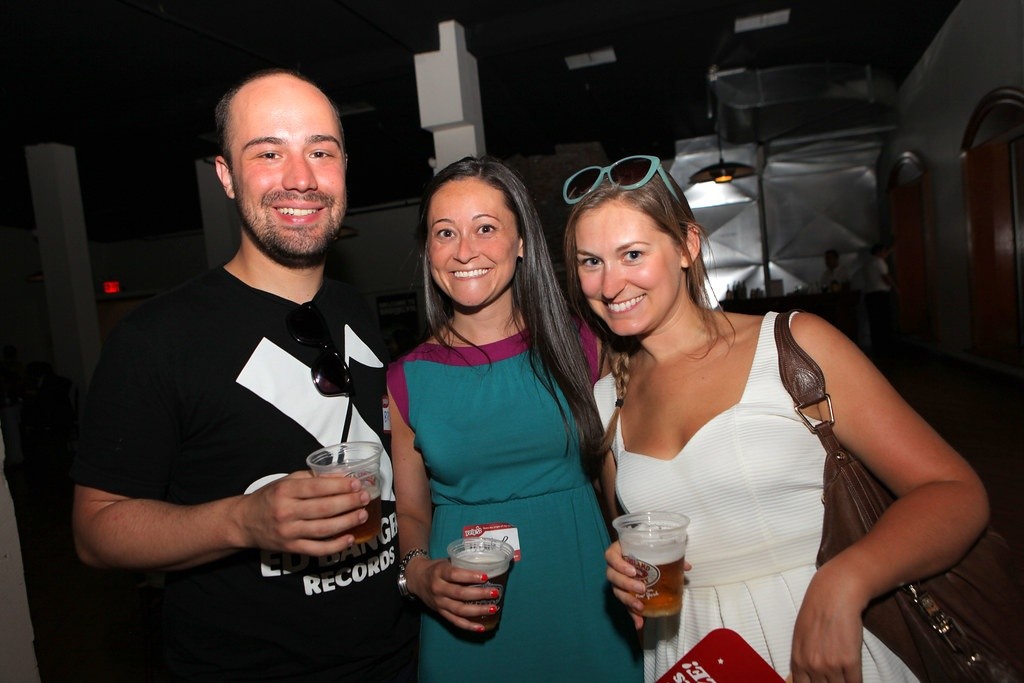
[613,511,690,618]
[446,538,514,631]
[307,441,384,544]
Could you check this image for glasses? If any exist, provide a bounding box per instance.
[287,300,355,397]
[563,155,688,215]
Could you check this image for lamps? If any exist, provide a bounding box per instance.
[688,94,756,184]
[330,225,359,244]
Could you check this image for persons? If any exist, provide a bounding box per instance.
[0,345,30,441]
[850,239,902,355]
[388,328,416,362]
[821,248,847,283]
[68,65,422,683]
[384,155,644,683]
[562,155,989,683]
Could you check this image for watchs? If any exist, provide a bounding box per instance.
[396,548,429,601]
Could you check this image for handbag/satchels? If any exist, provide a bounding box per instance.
[773,309,1024,683]
